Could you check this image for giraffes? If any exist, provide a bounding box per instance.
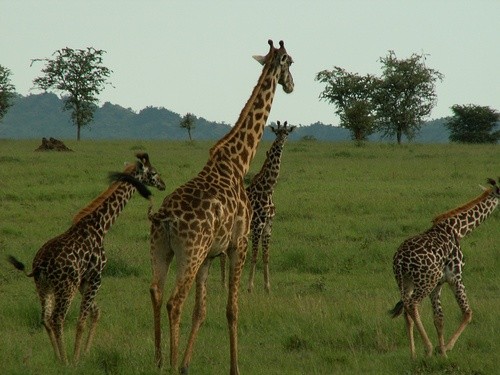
[146,37,297,375]
[6,150,169,370]
[219,120,296,294]
[386,177,500,364]
